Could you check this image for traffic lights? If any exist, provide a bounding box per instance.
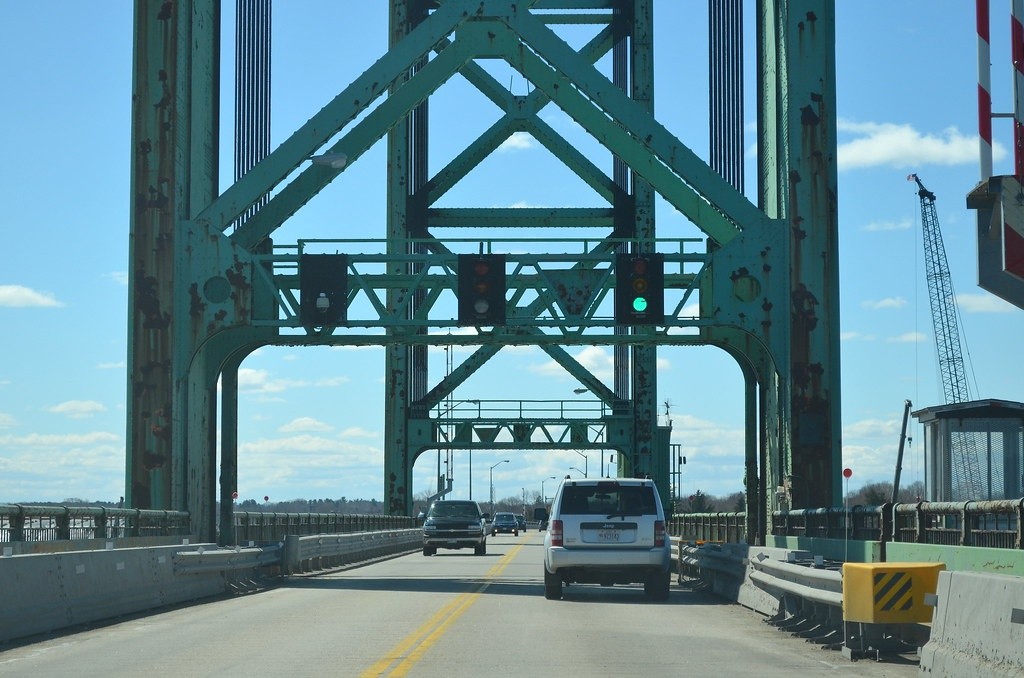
[458,254,507,328]
[615,252,664,326]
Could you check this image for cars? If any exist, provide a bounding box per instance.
[539,519,547,533]
[515,515,527,533]
[491,513,519,537]
[417,498,490,555]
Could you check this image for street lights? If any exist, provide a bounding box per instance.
[569,467,587,478]
[542,477,556,507]
[573,387,603,417]
[490,460,510,521]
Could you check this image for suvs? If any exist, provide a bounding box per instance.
[534,475,671,601]
